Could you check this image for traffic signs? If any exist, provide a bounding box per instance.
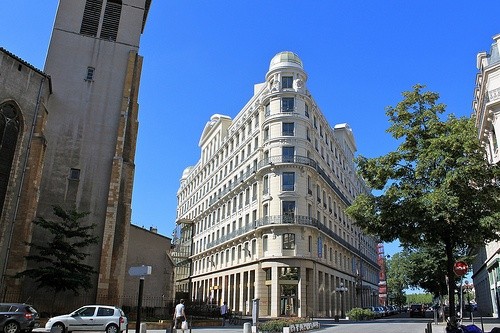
[128,266,152,276]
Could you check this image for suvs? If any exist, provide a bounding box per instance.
[45,305,127,333]
[410,304,426,318]
[0,303,41,333]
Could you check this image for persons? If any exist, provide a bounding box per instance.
[173,298,187,333]
[221,301,227,326]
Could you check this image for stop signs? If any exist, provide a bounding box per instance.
[453,261,468,276]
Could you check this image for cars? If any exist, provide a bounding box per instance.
[374,305,400,319]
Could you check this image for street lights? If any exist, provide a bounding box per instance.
[336,283,348,318]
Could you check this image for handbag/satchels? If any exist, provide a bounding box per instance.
[182,318,188,330]
